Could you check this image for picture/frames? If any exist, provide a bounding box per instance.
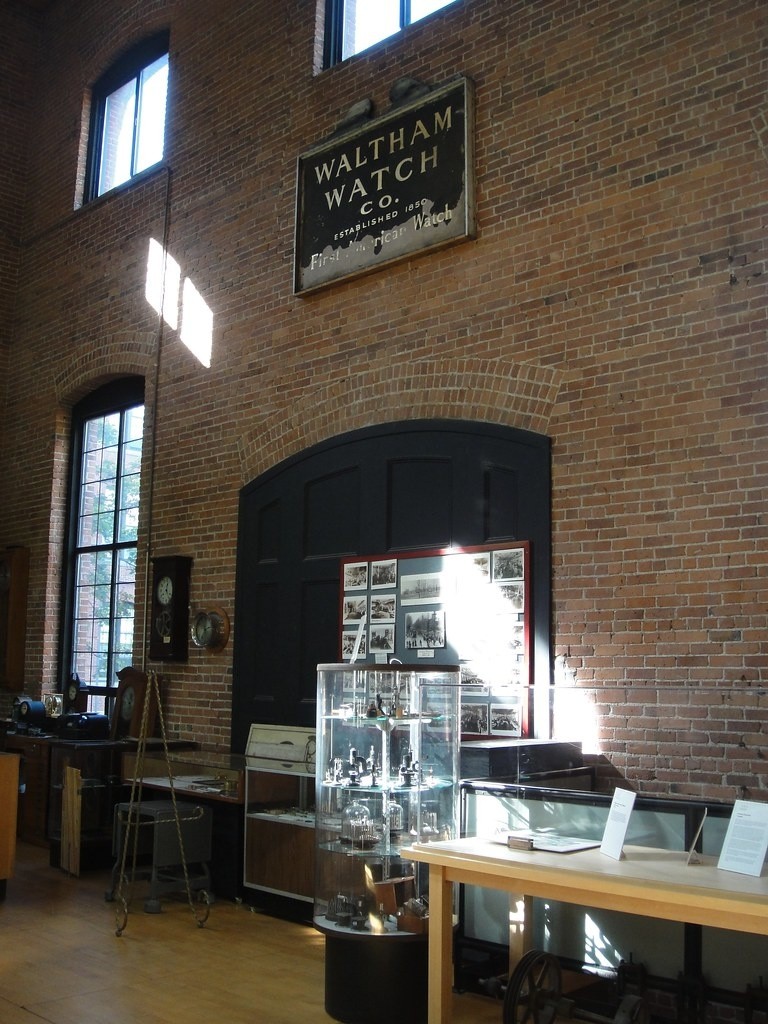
[338,539,532,741]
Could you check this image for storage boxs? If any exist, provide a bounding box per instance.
[375,875,429,935]
[245,723,316,774]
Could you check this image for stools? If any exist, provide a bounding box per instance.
[105,800,213,913]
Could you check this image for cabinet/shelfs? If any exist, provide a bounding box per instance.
[46,737,197,880]
[461,739,583,780]
[241,767,316,925]
[0,730,59,849]
[315,662,462,1024]
[0,545,30,690]
[0,752,21,903]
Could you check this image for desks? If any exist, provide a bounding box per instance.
[402,829,768,1024]
[123,774,244,903]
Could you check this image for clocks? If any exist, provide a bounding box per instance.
[148,555,191,664]
[18,701,45,729]
[109,666,161,742]
[190,605,229,654]
[43,693,63,718]
[12,696,32,723]
[64,673,89,714]
